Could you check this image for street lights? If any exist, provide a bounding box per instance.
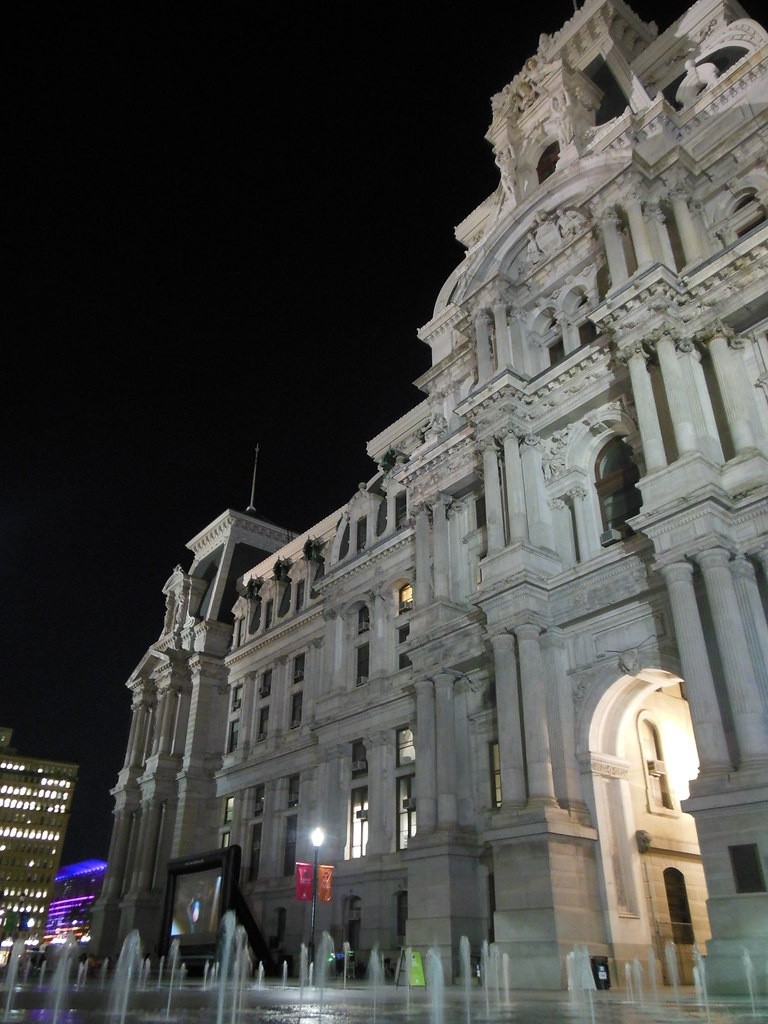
[308,826,324,970]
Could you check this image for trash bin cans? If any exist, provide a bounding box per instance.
[589,955,612,991]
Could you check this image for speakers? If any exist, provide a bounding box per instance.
[269,936,279,950]
[277,954,293,977]
